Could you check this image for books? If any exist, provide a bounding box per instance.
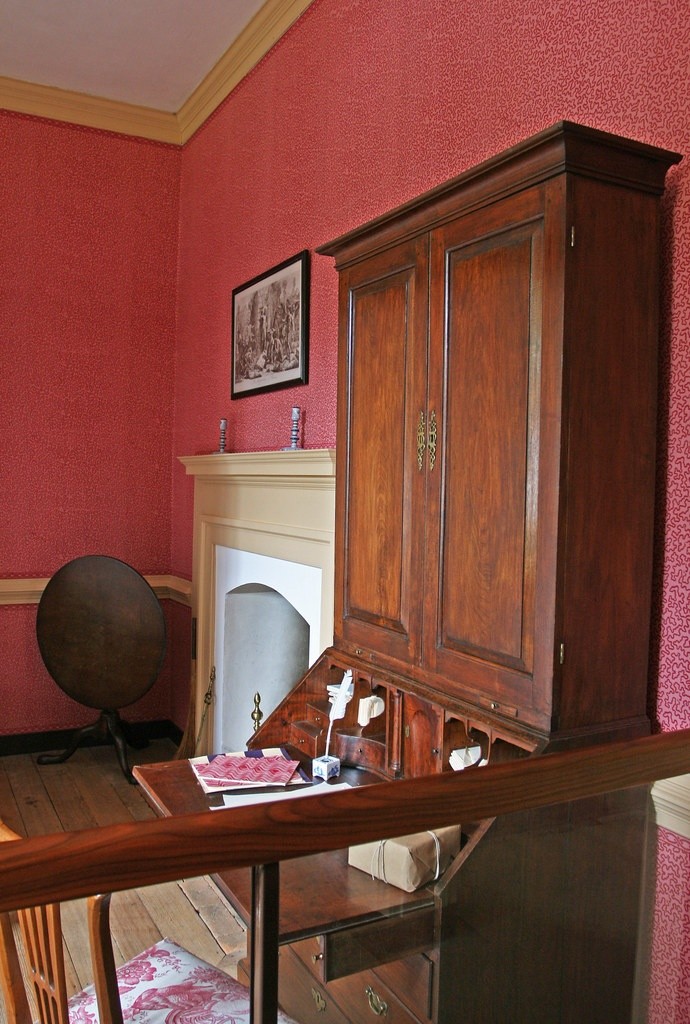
[188,747,313,794]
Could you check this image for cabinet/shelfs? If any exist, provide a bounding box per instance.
[133,118,683,1024]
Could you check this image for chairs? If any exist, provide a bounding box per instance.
[0,818,303,1024]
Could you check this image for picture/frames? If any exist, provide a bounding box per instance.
[230,248,310,401]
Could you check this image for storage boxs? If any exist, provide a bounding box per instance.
[348,824,461,893]
